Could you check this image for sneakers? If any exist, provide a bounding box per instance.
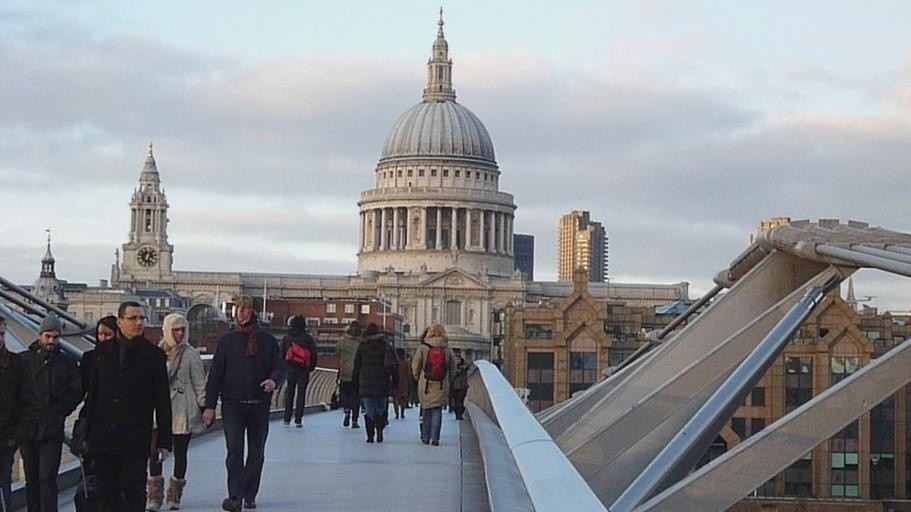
[243,496,257,510]
[421,437,440,446]
[220,497,243,512]
[281,420,303,429]
[341,415,360,429]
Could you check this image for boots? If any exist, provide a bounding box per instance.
[144,474,165,512]
[165,474,187,510]
[394,407,406,420]
[362,412,375,444]
[375,414,386,443]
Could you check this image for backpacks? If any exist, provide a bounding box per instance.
[420,342,448,396]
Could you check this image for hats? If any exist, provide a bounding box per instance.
[37,310,61,336]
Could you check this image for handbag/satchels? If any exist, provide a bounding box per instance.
[382,346,396,375]
[284,341,313,369]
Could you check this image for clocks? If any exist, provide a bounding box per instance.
[137,247,158,268]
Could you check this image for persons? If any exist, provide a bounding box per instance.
[279,315,317,427]
[336,320,469,446]
[0,294,174,512]
[146,294,285,512]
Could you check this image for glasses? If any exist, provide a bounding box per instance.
[121,315,145,321]
